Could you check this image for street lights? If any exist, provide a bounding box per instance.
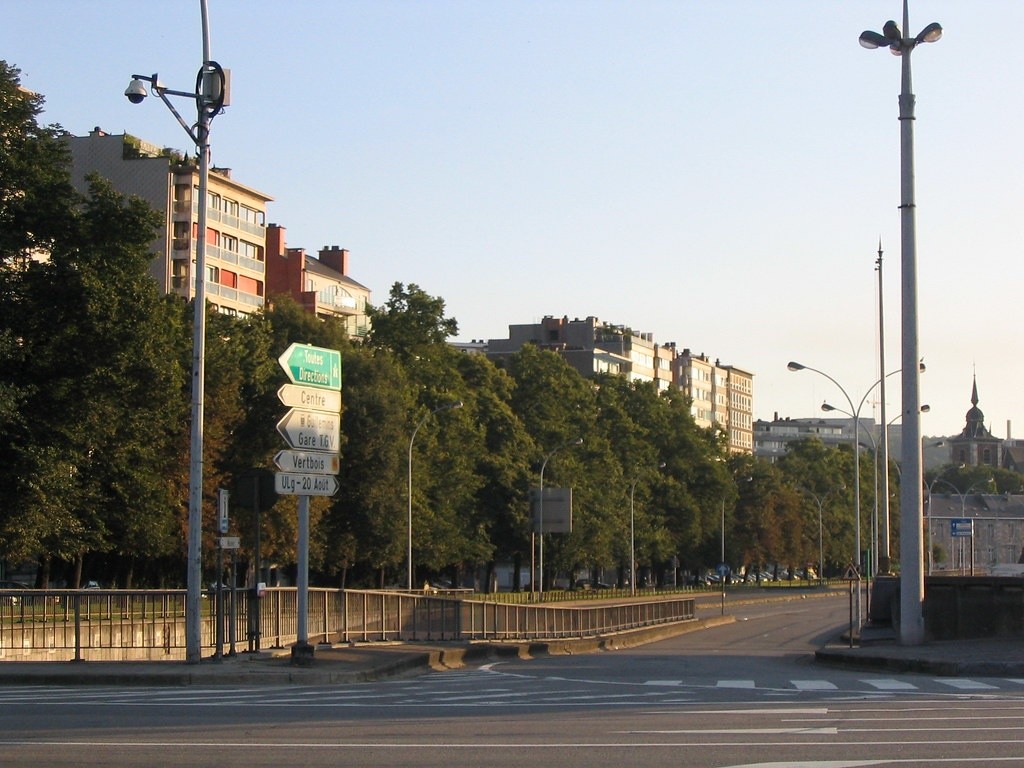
[721,475,754,618]
[857,0,944,646]
[818,402,930,573]
[933,475,996,569]
[125,0,231,667]
[785,359,927,639]
[404,402,465,592]
[629,461,667,597]
[798,485,846,587]
[538,437,584,598]
[921,463,965,573]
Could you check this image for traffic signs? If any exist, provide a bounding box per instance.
[277,343,342,390]
[276,407,340,453]
[273,449,339,474]
[276,384,341,413]
[274,472,340,497]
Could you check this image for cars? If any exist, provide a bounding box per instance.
[576,579,610,589]
[682,567,815,590]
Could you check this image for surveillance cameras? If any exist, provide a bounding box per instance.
[124,81,148,103]
